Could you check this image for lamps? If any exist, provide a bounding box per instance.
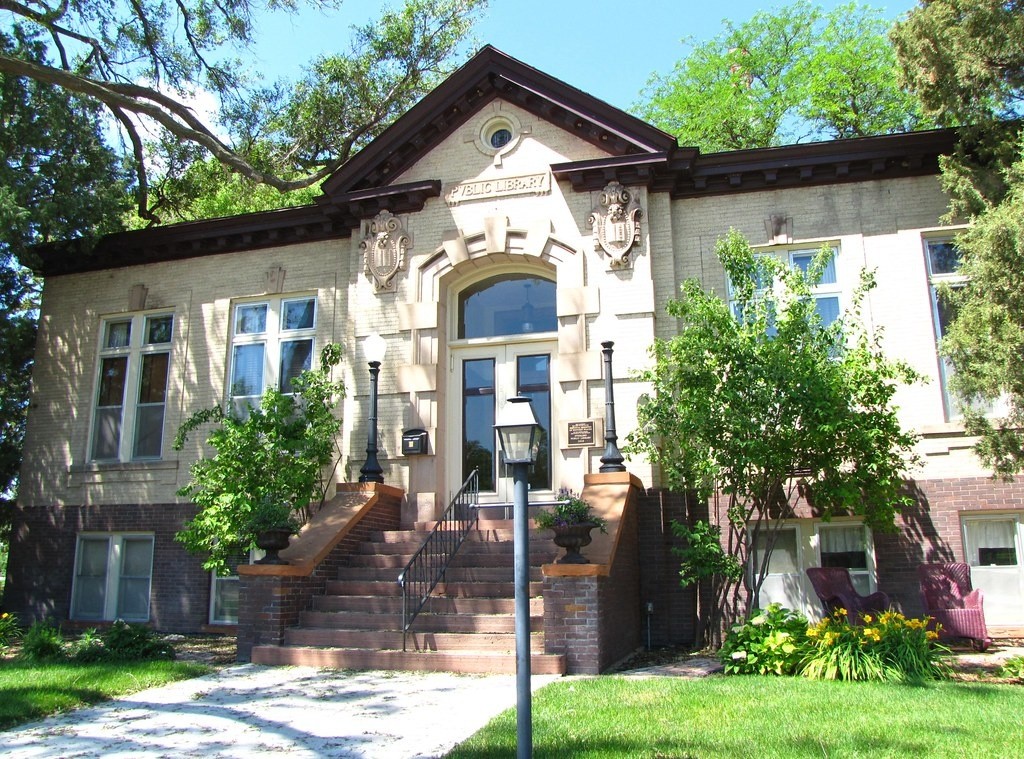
[521,284,536,333]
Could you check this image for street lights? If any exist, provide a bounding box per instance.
[595,311,626,472]
[491,390,545,759]
[359,332,387,483]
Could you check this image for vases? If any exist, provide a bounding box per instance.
[546,522,599,565]
[251,528,292,565]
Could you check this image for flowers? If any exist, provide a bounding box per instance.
[533,484,608,537]
[245,501,303,539]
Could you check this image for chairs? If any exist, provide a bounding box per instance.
[805,566,890,627]
[916,563,990,653]
